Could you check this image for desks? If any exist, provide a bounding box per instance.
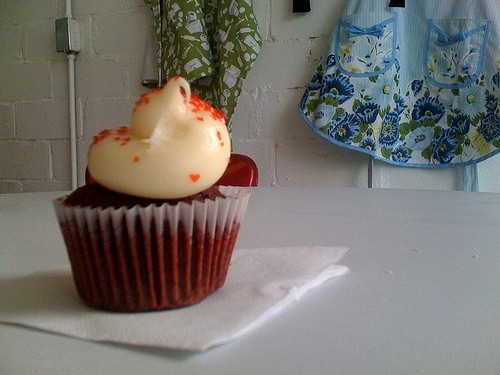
[0,192,498,373]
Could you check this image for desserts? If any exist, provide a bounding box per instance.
[51,74,250,312]
